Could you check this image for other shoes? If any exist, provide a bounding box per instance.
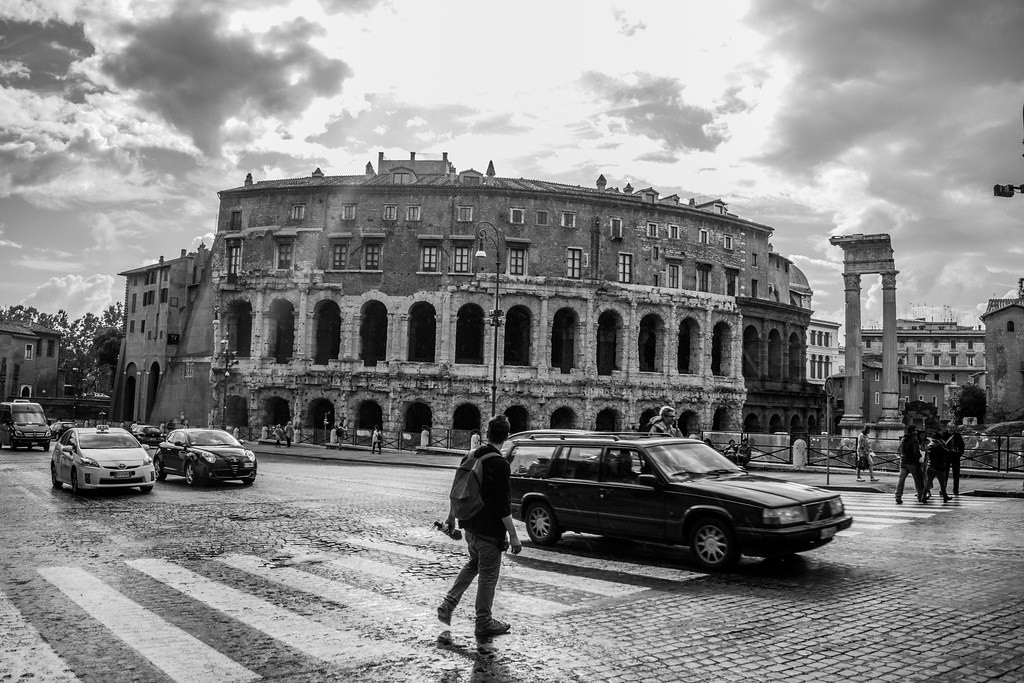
[921,497,928,503]
[895,498,903,504]
[870,477,879,481]
[856,478,865,482]
[943,494,953,503]
[918,495,923,502]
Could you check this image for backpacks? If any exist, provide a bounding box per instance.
[448,449,501,520]
[335,428,344,437]
[377,432,383,440]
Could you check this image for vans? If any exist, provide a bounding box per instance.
[0,400,51,451]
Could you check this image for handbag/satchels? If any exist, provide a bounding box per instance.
[858,457,869,470]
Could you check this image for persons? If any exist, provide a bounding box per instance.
[895,422,964,504]
[84,419,383,454]
[437,415,522,637]
[856,424,879,482]
[647,407,751,468]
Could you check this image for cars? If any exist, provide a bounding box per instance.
[131,425,165,447]
[50,421,77,440]
[50,425,157,497]
[152,428,258,487]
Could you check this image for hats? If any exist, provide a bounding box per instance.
[742,437,747,442]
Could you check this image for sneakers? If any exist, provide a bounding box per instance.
[474,619,510,636]
[437,605,452,627]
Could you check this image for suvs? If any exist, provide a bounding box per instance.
[501,428,853,573]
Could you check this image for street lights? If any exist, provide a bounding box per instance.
[473,219,502,418]
